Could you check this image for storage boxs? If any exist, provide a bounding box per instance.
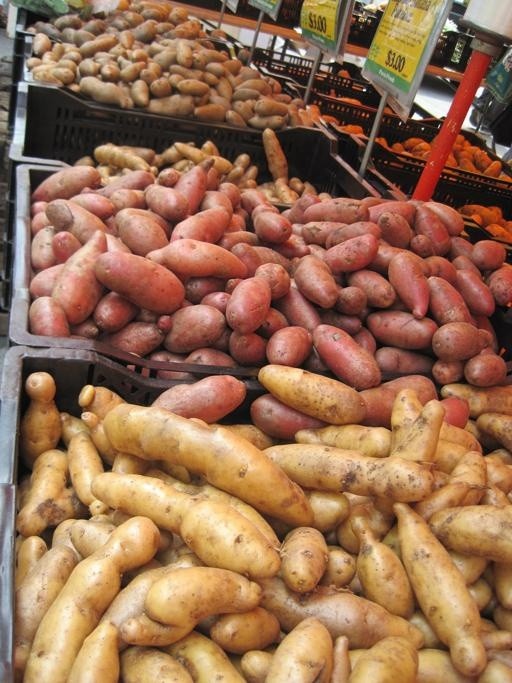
[8,81,383,209]
[365,113,511,211]
[289,77,444,176]
[14,7,259,98]
[1,345,511,677]
[13,162,509,378]
[249,58,441,136]
[25,35,335,146]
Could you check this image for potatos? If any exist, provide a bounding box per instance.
[27,157,512,440]
[75,128,334,203]
[24,1,314,128]
[307,103,512,193]
[11,362,512,683]
